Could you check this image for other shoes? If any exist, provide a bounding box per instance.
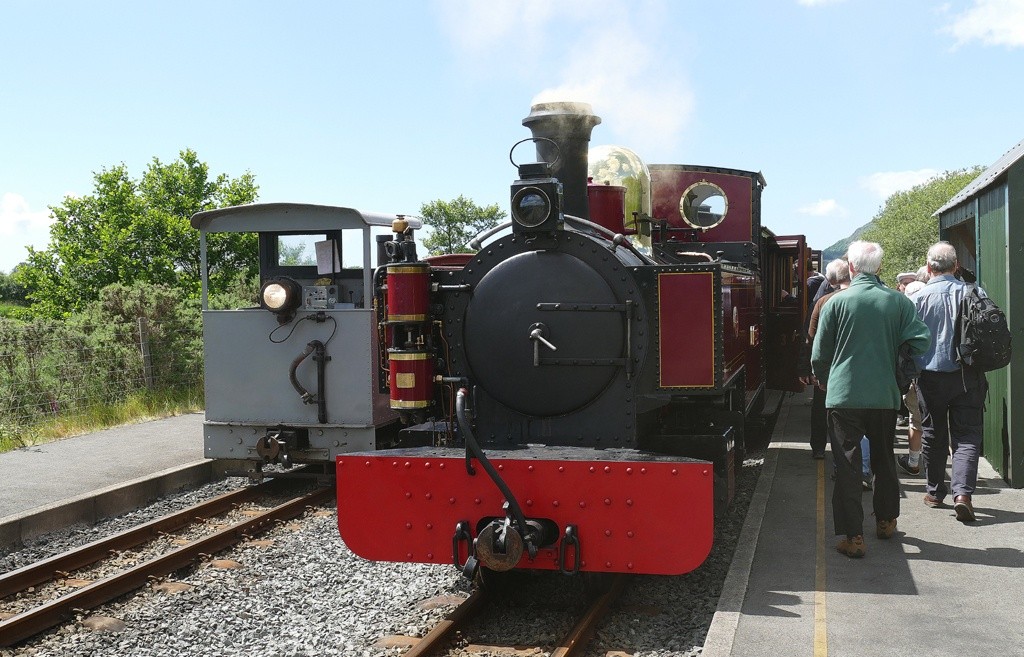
[898,454,920,476]
[862,474,873,490]
[812,450,825,458]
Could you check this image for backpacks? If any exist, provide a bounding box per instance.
[956,284,1012,372]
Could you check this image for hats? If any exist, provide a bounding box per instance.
[897,273,916,282]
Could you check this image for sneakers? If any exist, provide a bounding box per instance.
[837,535,865,558]
[876,518,898,538]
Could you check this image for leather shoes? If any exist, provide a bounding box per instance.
[954,496,976,521]
[923,494,943,508]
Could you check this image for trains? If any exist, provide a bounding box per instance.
[190,98,825,598]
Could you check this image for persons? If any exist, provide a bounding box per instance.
[809,241,932,558]
[914,240,988,520]
[793,258,980,476]
